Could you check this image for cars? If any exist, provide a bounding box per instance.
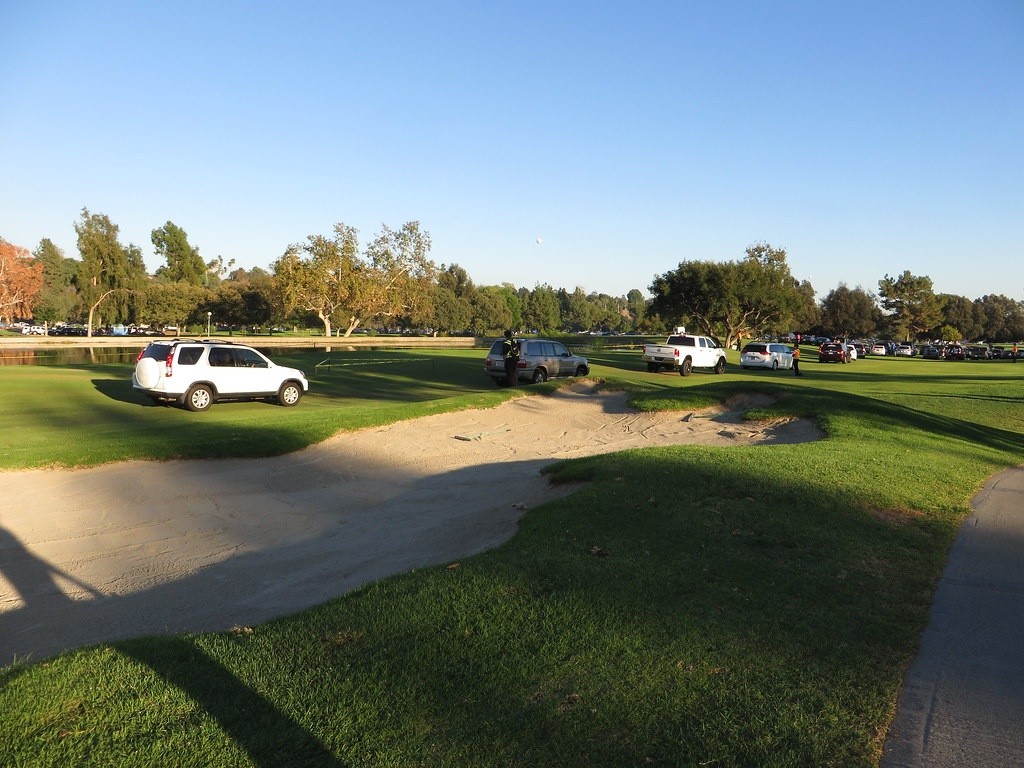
[919,344,1024,360]
[739,342,794,371]
[847,345,859,361]
[20,324,87,337]
[763,332,830,345]
[896,344,914,357]
[870,345,886,356]
[854,343,866,359]
[818,342,851,365]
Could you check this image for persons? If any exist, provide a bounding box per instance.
[736,329,742,351]
[888,340,901,356]
[796,333,800,344]
[925,338,961,345]
[792,343,800,376]
[502,329,519,386]
[1012,343,1018,363]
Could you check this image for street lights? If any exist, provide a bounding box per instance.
[206,312,212,336]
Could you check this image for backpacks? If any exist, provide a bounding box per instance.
[510,341,520,362]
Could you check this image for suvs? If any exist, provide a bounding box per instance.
[483,337,591,386]
[131,338,308,413]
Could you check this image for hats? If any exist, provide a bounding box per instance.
[504,330,512,336]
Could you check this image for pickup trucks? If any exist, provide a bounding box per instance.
[642,333,727,377]
